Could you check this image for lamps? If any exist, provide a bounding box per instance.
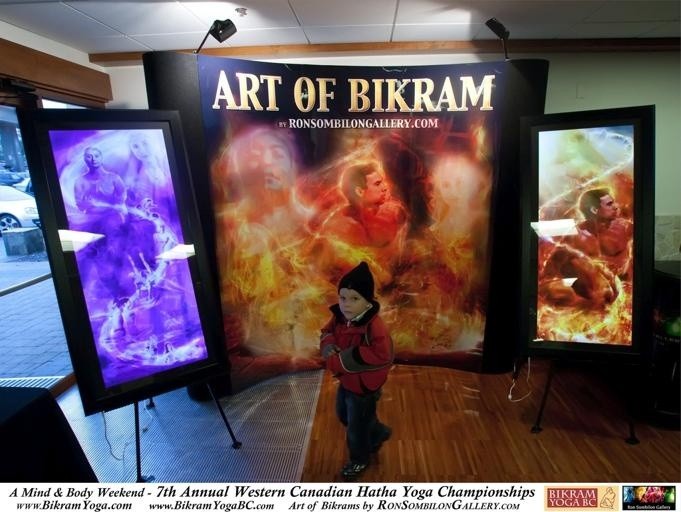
[194,18,237,54]
[484,16,510,61]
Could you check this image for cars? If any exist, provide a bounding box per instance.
[0,160,44,239]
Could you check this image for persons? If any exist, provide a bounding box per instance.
[223,126,319,232]
[74,145,141,340]
[538,187,636,310]
[320,260,397,479]
[425,147,489,319]
[320,160,408,245]
[122,130,203,336]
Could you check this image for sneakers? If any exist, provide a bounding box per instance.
[339,456,370,480]
[370,421,392,455]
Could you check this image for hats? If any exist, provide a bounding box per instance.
[338,261,374,301]
